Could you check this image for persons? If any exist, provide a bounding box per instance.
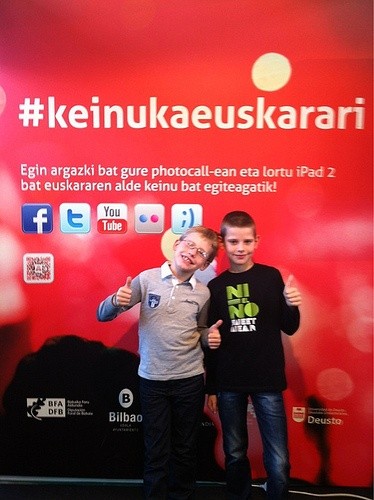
[98,225,223,500]
[203,211,301,500]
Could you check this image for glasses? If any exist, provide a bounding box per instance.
[180,240,210,263]
